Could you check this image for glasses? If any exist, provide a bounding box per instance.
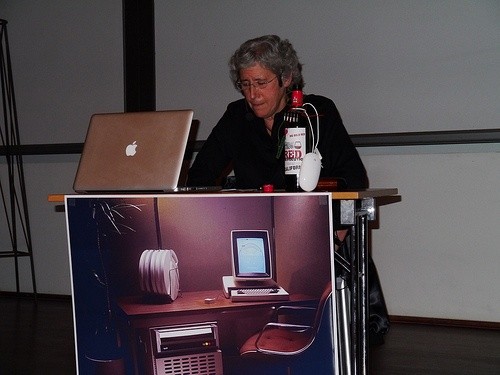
[234,75,279,90]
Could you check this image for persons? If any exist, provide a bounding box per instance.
[183,34,369,251]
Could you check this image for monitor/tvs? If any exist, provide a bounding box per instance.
[231,229,273,281]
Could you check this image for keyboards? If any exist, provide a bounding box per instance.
[237,288,278,294]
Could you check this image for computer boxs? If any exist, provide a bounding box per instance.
[137,321,224,375]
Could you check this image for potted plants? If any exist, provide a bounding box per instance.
[83,202,142,375]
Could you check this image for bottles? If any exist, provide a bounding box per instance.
[283,84,312,193]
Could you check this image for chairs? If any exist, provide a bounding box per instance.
[238,282,332,374]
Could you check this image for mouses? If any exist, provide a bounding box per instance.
[299,152,321,192]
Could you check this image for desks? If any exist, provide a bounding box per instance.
[108,282,321,374]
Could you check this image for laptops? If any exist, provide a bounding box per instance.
[72,109,223,194]
